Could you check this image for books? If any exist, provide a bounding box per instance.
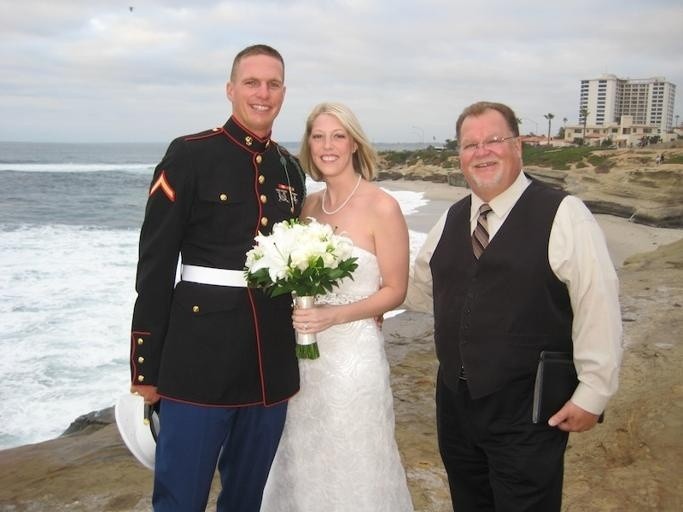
[532,351,604,424]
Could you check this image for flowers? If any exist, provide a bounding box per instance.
[242,214,358,360]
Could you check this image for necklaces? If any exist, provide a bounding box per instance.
[321,173,361,215]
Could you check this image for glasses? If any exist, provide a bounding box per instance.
[456,135,515,154]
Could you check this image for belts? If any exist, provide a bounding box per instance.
[182,264,273,288]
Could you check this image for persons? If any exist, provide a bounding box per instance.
[129,45,383,512]
[260,102,414,512]
[392,101,622,512]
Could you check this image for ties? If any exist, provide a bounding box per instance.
[471,204,492,260]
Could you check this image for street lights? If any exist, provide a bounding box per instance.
[544,114,553,145]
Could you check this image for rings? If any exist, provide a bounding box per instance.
[304,323,308,330]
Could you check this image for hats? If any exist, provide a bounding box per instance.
[114,392,160,470]
